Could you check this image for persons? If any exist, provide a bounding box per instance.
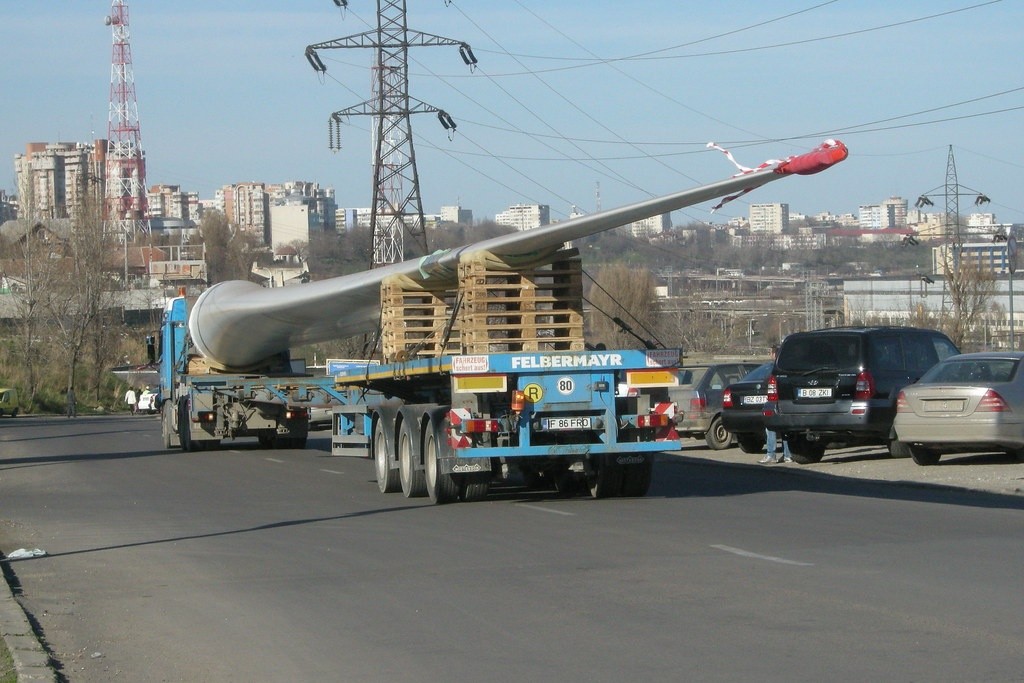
[66,386,78,419]
[124,384,151,417]
[758,348,794,465]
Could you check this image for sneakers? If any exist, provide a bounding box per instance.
[777,455,793,463]
[756,455,777,464]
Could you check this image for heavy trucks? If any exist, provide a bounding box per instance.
[144,294,683,505]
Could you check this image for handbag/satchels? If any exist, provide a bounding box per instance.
[135,405,139,413]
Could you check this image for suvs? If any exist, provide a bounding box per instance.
[761,324,992,464]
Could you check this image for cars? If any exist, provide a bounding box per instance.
[721,362,775,453]
[0,388,19,418]
[308,391,333,430]
[138,386,160,415]
[893,350,1024,466]
[667,359,771,451]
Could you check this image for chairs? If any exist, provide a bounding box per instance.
[962,362,1007,380]
